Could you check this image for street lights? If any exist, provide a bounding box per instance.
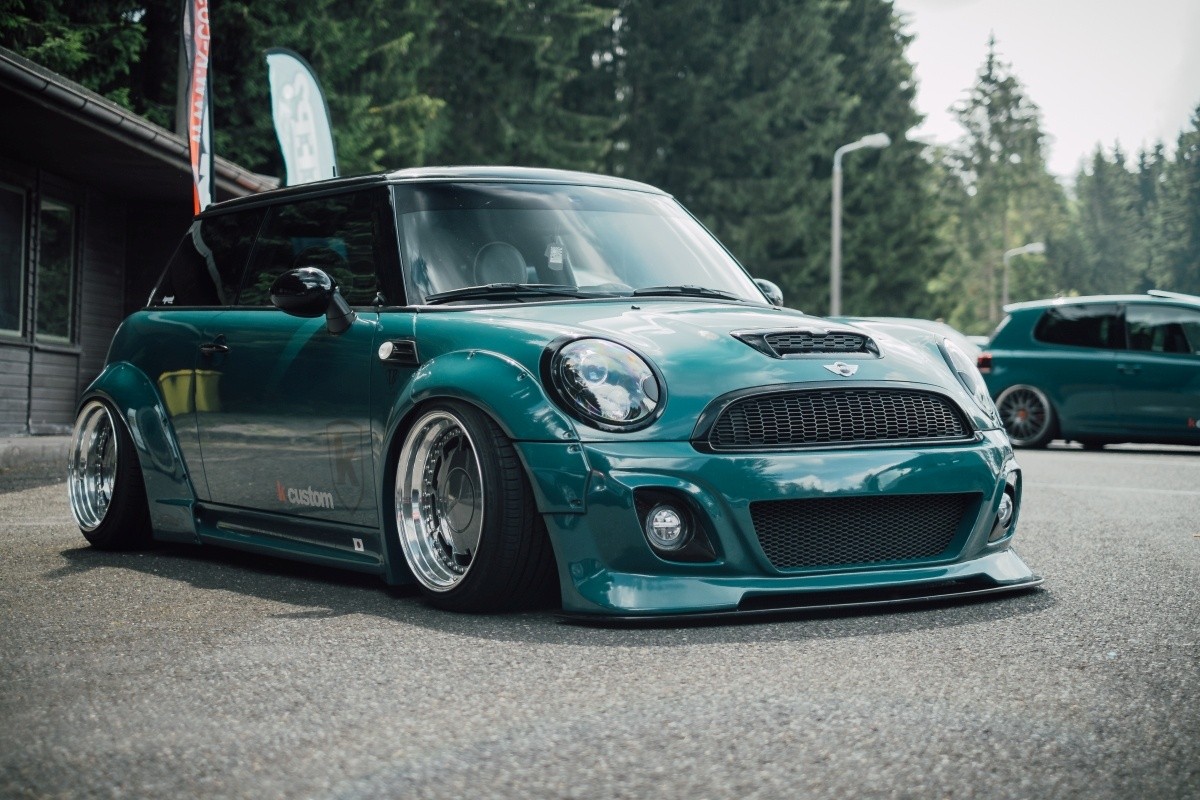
[829,131,893,318]
[1002,241,1046,304]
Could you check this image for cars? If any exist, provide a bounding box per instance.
[67,159,1042,625]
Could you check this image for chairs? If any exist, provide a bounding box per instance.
[471,241,535,285]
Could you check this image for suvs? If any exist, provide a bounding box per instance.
[975,289,1200,450]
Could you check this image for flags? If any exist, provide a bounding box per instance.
[171,1,219,223]
[262,44,341,193]
